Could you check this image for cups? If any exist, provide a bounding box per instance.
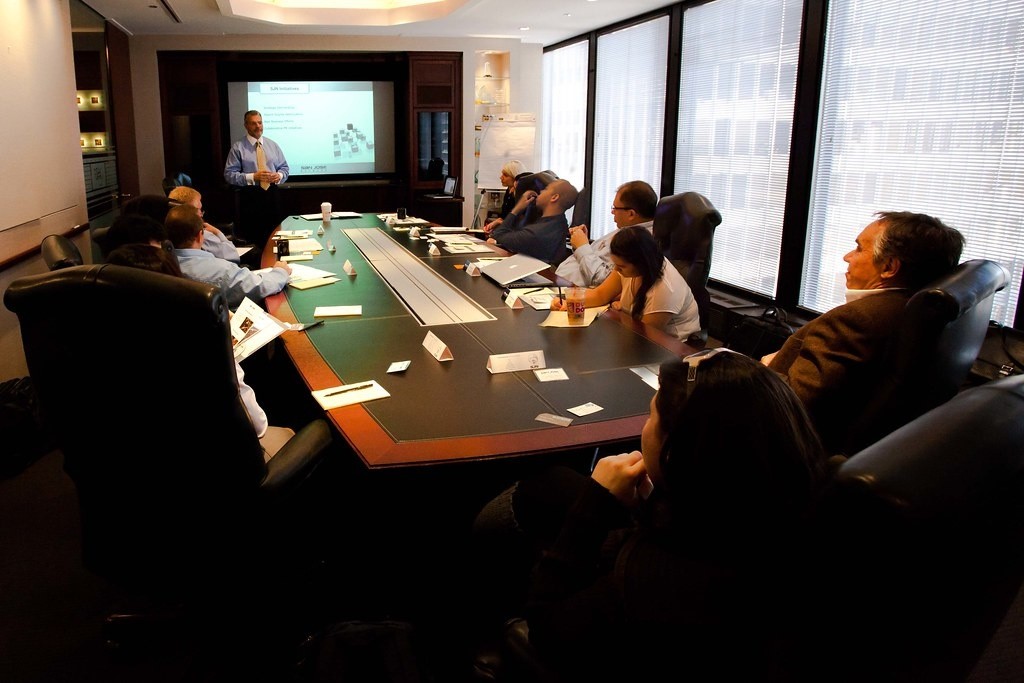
[277,238,290,261]
[321,203,332,221]
[564,287,585,325]
[397,208,406,219]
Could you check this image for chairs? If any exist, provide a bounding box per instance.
[4,172,1024,683]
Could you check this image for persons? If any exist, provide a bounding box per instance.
[483,158,577,266]
[225,111,290,255]
[476,351,899,683]
[550,226,702,345]
[102,184,291,437]
[765,210,965,456]
[555,181,657,290]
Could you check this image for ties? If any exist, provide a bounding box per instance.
[255,139,271,191]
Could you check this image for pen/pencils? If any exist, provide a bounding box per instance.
[298,319,325,332]
[558,286,562,306]
[292,217,299,219]
[452,243,472,245]
[325,384,373,397]
[482,259,503,261]
[486,223,487,230]
[524,287,545,295]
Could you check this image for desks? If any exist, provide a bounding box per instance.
[82,150,121,220]
[260,212,701,477]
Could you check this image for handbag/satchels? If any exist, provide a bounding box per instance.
[722,305,795,363]
[969,320,1024,384]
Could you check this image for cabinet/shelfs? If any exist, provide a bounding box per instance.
[409,51,465,226]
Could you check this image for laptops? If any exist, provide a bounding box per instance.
[424,175,458,198]
[478,253,554,287]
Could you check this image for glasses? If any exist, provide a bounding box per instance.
[682,346,754,401]
[195,207,205,217]
[611,204,631,211]
